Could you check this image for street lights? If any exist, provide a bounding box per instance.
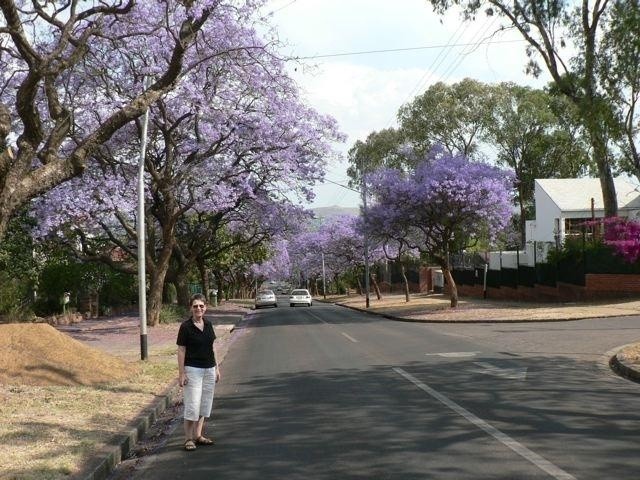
[134,90,176,362]
[306,171,370,310]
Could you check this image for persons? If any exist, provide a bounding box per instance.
[175,292,221,451]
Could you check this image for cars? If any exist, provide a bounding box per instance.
[288,288,313,308]
[254,289,278,309]
[270,281,291,295]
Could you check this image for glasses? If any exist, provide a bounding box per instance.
[193,304,205,309]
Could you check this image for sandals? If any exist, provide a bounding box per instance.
[184,438,196,451]
[195,436,213,444]
